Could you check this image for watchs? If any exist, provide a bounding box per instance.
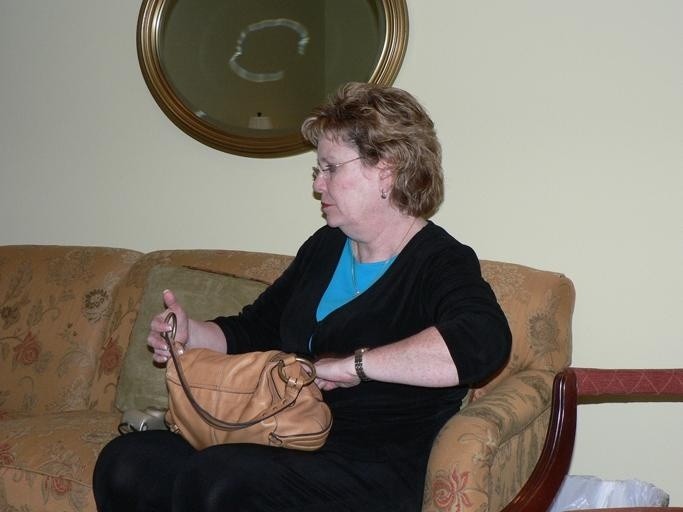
[355,347,371,382]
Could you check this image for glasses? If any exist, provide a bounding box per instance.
[312,154,369,178]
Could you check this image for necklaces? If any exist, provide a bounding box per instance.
[353,219,416,295]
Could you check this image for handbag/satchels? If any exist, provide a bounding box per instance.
[160,310,335,451]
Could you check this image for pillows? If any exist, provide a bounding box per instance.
[113,262,269,413]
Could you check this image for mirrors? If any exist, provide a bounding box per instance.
[136,0,411,160]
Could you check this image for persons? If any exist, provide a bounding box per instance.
[92,82,512,512]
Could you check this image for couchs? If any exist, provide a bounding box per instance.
[0,246,576,512]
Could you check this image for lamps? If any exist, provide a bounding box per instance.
[247,112,275,130]
